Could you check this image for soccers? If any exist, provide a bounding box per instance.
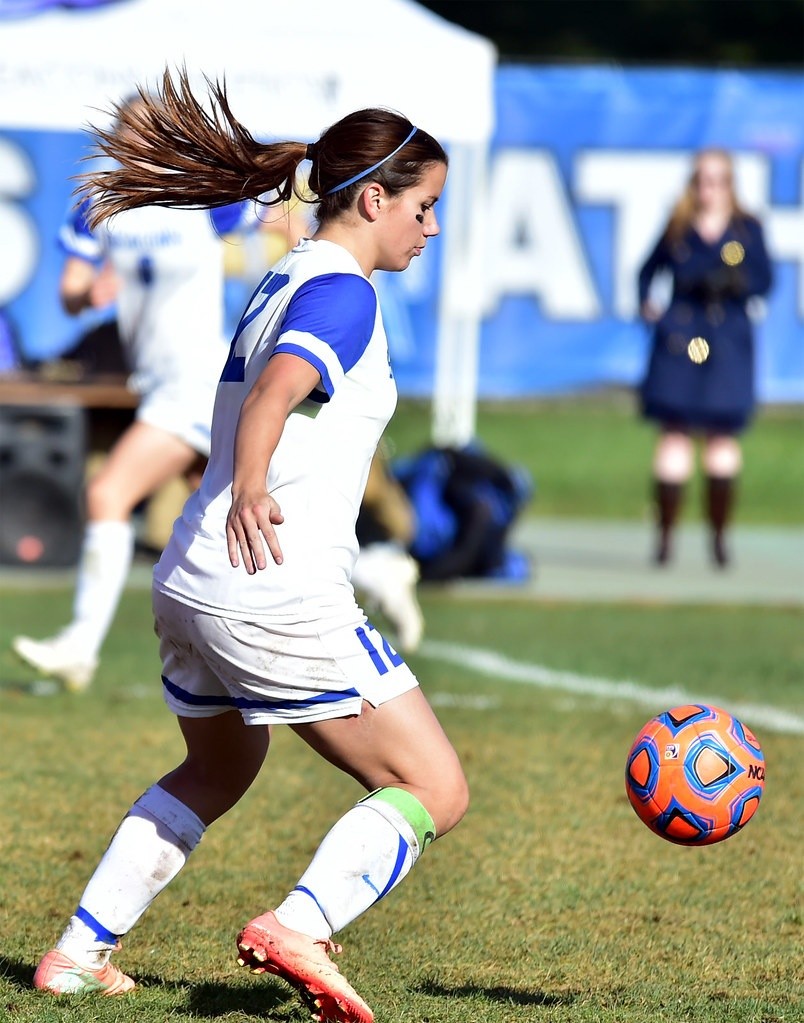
[621,702,768,848]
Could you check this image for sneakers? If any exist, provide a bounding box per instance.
[236,910,374,1023]
[34,940,136,997]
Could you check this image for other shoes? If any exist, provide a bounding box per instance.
[352,541,425,655]
[9,635,98,694]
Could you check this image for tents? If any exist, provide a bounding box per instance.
[0,0,497,450]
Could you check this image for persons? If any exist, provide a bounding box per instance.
[35,66,470,1023]
[8,94,304,699]
[629,148,772,570]
